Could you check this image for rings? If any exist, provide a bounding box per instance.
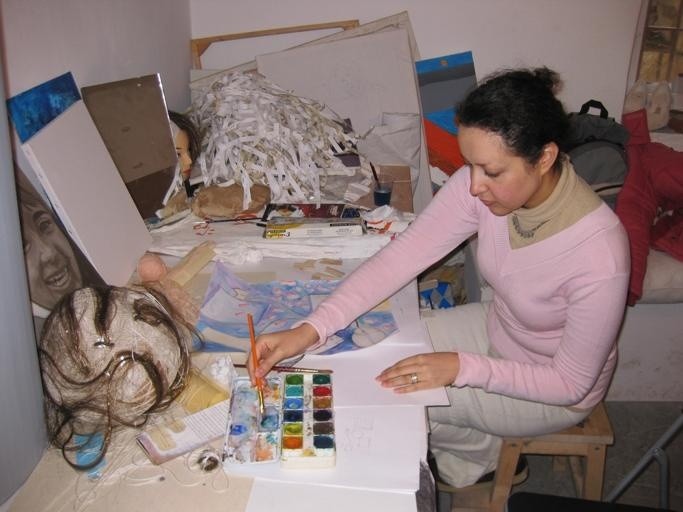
[409,372,418,384]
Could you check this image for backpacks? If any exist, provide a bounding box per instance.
[558,99,629,214]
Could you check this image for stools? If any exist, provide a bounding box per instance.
[451,401,615,512]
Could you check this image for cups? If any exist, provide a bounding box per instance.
[371,174,395,207]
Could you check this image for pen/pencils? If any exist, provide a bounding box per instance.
[205,218,262,223]
[369,162,381,190]
[256,223,266,227]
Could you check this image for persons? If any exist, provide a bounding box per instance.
[17,178,85,351]
[242,68,631,490]
[165,109,200,186]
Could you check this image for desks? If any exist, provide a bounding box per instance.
[0,241,417,511]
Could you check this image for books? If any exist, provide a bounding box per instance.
[257,200,369,240]
[8,69,182,291]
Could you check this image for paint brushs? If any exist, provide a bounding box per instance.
[232,313,333,420]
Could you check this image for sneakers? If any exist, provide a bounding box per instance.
[428,454,530,493]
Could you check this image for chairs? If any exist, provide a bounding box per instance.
[503,413,683,512]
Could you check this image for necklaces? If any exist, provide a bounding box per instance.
[509,209,551,242]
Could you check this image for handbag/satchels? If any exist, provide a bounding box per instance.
[622,78,672,131]
